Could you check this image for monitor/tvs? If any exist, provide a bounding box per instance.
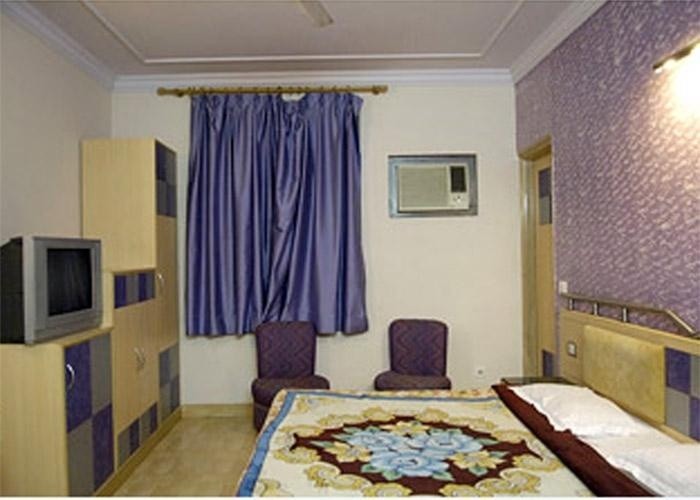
[0,235,103,347]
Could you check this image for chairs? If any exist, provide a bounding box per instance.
[250,319,330,433]
[375,317,452,392]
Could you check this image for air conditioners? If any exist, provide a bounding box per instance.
[394,161,471,212]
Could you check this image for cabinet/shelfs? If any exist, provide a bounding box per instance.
[1,137,184,495]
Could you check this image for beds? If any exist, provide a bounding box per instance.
[232,291,699,498]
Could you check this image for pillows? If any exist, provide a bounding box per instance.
[508,385,700,499]
